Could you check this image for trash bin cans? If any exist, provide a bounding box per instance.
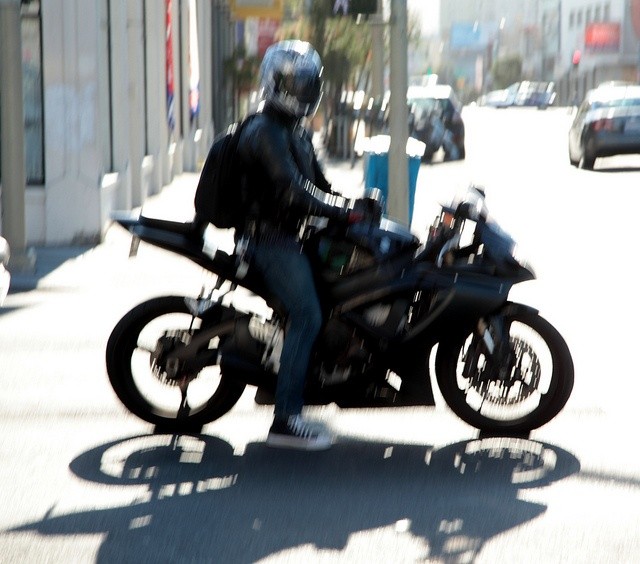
[362,133,426,230]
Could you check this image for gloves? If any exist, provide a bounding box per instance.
[352,197,383,224]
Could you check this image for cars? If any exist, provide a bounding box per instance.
[569,86,640,169]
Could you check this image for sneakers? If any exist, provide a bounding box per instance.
[267,414,331,451]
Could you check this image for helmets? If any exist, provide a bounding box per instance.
[258,40,323,119]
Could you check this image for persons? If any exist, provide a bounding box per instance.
[193,38,378,454]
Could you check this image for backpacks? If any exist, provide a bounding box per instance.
[193,109,301,230]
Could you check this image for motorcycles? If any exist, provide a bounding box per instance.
[106,186,574,432]
